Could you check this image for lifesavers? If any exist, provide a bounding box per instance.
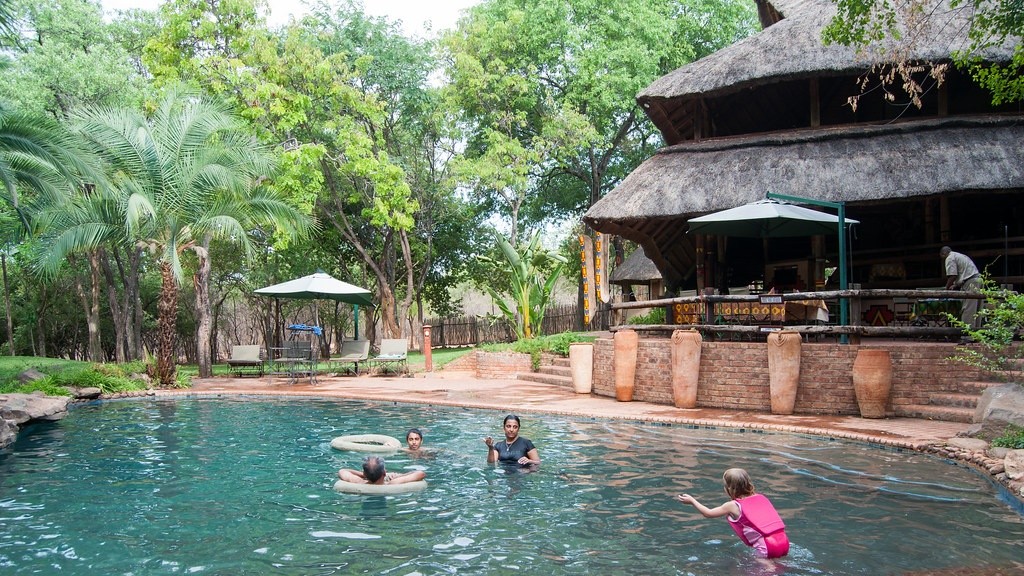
[330,434,402,452]
[334,471,428,494]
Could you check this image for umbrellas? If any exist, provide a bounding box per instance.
[686,197,860,291]
[253,269,376,327]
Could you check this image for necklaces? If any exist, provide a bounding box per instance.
[506,436,518,451]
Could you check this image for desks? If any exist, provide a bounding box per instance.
[268,347,318,386]
[893,299,989,341]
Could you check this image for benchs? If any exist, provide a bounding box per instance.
[329,340,369,377]
[226,345,264,378]
[367,339,408,376]
[274,341,311,378]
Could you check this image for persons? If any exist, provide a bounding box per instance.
[400,428,429,453]
[941,247,984,345]
[678,469,790,559]
[338,456,426,485]
[482,415,540,465]
